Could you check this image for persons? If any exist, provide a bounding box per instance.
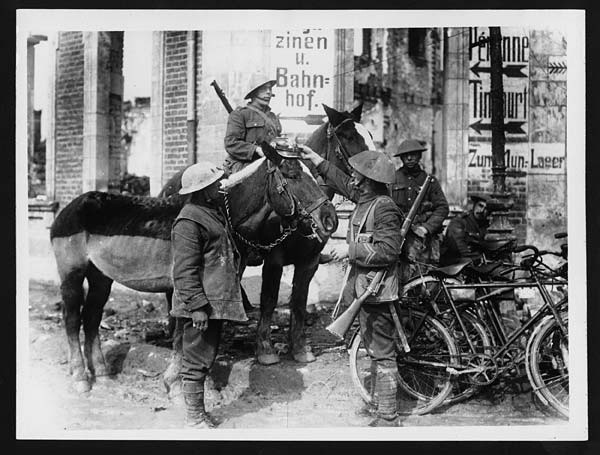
[225,70,282,173]
[296,142,403,428]
[440,195,513,265]
[168,161,247,430]
[395,140,447,265]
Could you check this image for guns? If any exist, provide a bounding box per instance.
[328,172,435,341]
[207,80,233,116]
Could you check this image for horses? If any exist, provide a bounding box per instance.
[50,135,338,393]
[156,101,376,365]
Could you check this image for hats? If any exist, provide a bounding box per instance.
[472,195,491,204]
[244,72,277,98]
[394,141,427,157]
[179,162,224,195]
[349,150,397,184]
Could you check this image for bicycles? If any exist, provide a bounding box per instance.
[394,232,568,402]
[345,245,568,418]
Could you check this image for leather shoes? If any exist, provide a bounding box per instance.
[355,405,377,417]
[369,418,399,427]
[186,416,217,429]
